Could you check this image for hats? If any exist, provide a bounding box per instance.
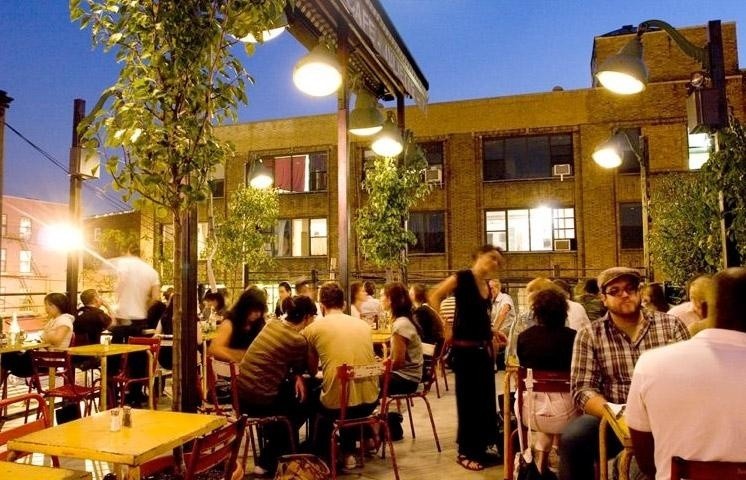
[597,265,641,291]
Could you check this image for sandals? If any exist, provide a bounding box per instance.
[456,454,485,471]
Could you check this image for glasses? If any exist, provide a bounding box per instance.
[605,284,640,297]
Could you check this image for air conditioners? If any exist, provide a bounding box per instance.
[553,240,571,252]
[553,164,571,176]
[424,168,442,182]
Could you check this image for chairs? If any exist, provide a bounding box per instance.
[0,312,746,479]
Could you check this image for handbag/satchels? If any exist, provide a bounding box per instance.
[206,377,234,405]
[420,341,436,364]
[376,411,404,442]
[514,447,559,480]
[272,451,330,480]
[56,402,82,428]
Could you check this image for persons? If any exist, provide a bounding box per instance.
[0,238,745,479]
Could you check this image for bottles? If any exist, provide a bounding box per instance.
[122,404,132,426]
[109,411,120,431]
[100,330,113,347]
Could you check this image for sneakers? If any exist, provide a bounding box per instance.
[341,451,358,469]
